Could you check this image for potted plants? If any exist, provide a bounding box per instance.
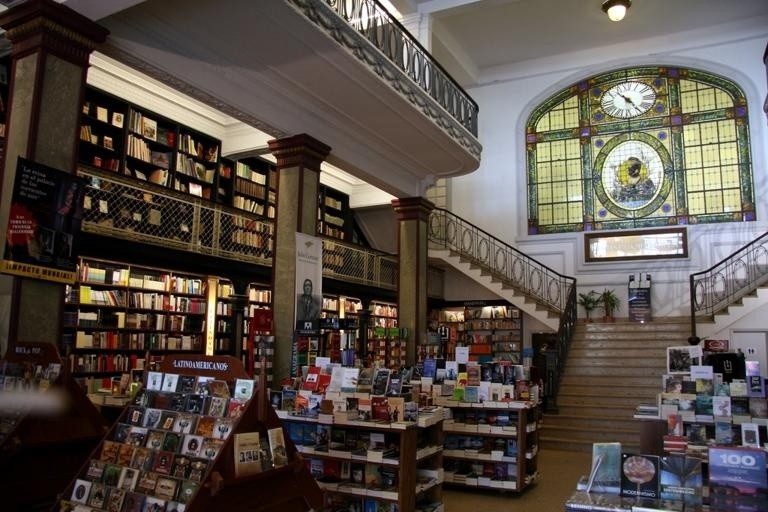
[577,290,602,323]
[596,288,622,322]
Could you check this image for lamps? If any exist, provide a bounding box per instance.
[601,0,632,22]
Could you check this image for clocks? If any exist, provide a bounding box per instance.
[600,81,656,119]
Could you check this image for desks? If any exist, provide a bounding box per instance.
[564,476,711,512]
[633,404,768,457]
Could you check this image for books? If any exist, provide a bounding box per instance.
[78,103,344,267]
[576,340,768,512]
[1,262,543,512]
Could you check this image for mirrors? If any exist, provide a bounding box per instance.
[584,227,688,263]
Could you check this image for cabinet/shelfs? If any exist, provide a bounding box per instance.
[323,293,524,382]
[79,255,273,395]
[77,85,350,276]
[78,377,328,512]
[265,402,441,510]
[428,402,537,498]
